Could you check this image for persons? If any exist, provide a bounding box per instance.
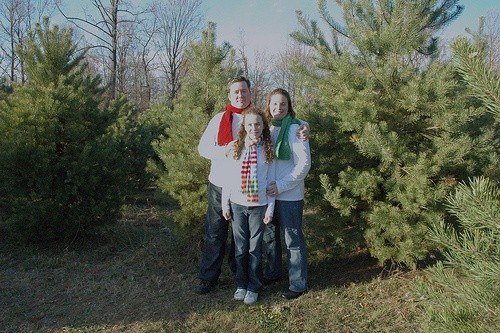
[260,87,312,299]
[197,75,309,295]
[221,107,276,304]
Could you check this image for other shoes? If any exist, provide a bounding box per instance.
[234,287,247,301]
[282,287,306,299]
[243,289,258,304]
[262,273,282,285]
[196,276,219,294]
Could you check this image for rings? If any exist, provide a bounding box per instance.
[273,192,275,195]
